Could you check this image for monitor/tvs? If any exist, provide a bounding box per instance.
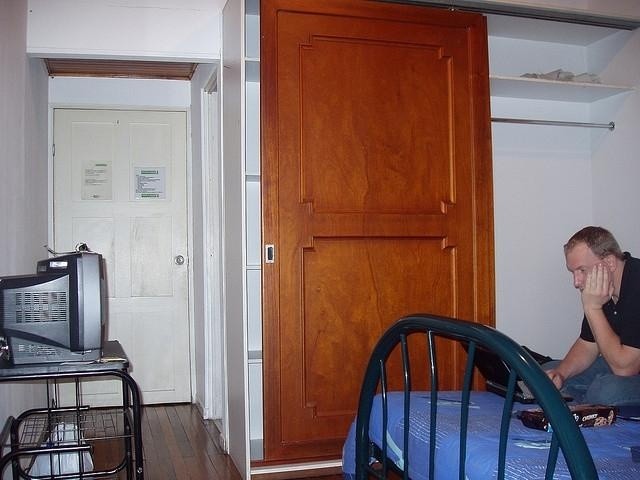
[0,251,109,365]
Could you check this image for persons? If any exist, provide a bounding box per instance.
[541,226,640,406]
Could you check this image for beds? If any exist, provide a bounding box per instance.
[355,313,640,478]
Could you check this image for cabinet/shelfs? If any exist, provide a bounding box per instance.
[1,338,145,480]
[221,0,638,480]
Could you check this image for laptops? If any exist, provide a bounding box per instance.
[460,342,575,404]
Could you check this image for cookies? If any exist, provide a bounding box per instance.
[594,417,607,426]
[607,410,615,425]
